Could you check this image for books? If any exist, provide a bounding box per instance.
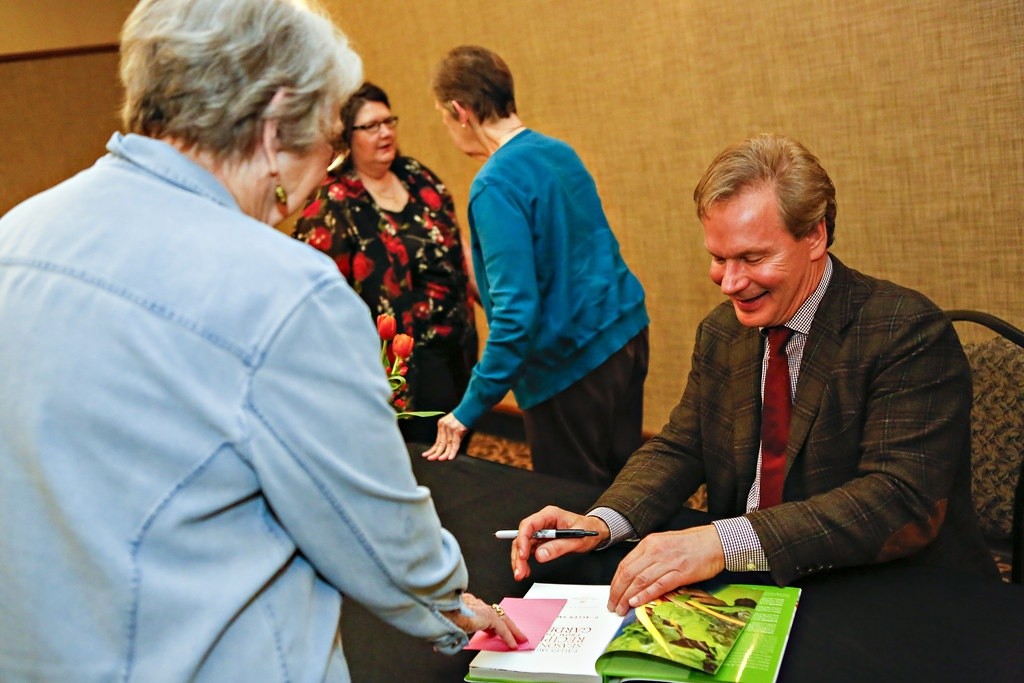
[461,581,802,683]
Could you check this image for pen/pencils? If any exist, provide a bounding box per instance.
[493,529,599,538]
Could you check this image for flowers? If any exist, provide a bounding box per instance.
[376,313,444,420]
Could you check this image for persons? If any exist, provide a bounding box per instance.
[511,133,1024,683]
[292,78,484,457]
[0,0,527,683]
[423,40,651,498]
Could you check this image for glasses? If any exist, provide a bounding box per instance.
[352,116,398,132]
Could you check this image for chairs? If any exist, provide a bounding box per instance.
[943,307,1023,584]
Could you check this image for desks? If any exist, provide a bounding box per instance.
[340,441,1024,683]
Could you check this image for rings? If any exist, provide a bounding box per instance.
[492,603,505,615]
[446,440,452,445]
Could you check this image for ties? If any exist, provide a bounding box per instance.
[760,327,795,509]
